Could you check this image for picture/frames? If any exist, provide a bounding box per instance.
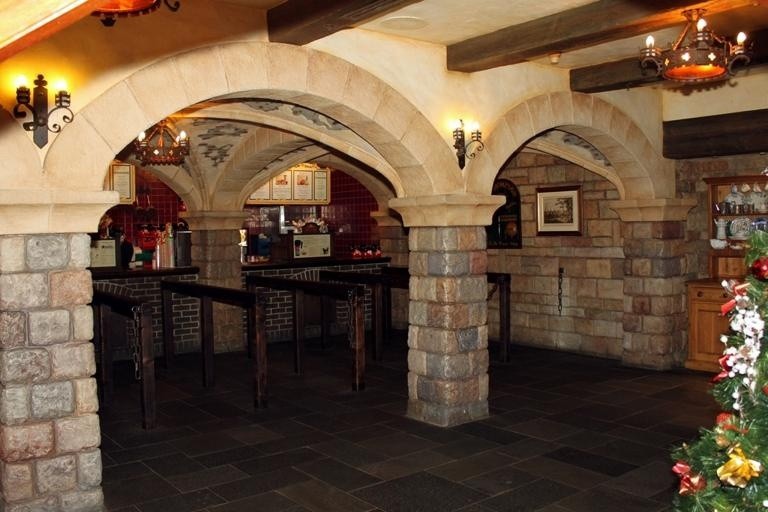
[535,185,584,237]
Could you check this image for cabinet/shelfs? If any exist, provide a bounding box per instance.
[685,175,766,375]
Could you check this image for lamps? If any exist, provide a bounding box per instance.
[448,118,485,170]
[11,74,74,149]
[637,8,755,86]
[133,115,190,167]
